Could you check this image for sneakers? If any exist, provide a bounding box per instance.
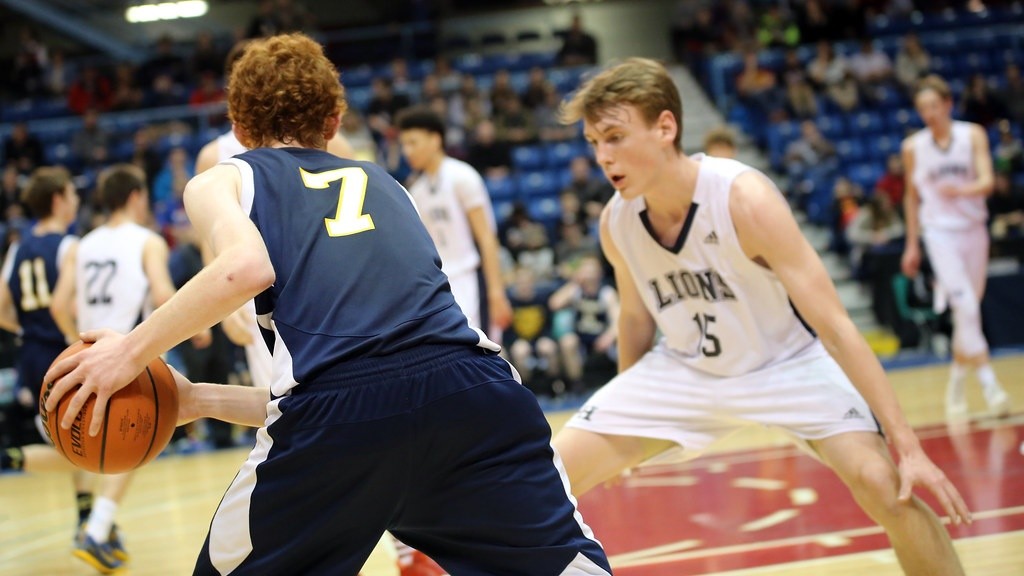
[75,522,127,560]
[73,532,127,576]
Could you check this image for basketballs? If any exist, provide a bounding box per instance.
[39,339,180,476]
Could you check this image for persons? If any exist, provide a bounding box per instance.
[657,0,1024,352]
[396,110,513,335]
[42,31,612,576]
[900,75,1009,426]
[552,55,973,576]
[0,13,623,576]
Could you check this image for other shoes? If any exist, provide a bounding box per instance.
[945,382,970,412]
[982,380,1007,409]
